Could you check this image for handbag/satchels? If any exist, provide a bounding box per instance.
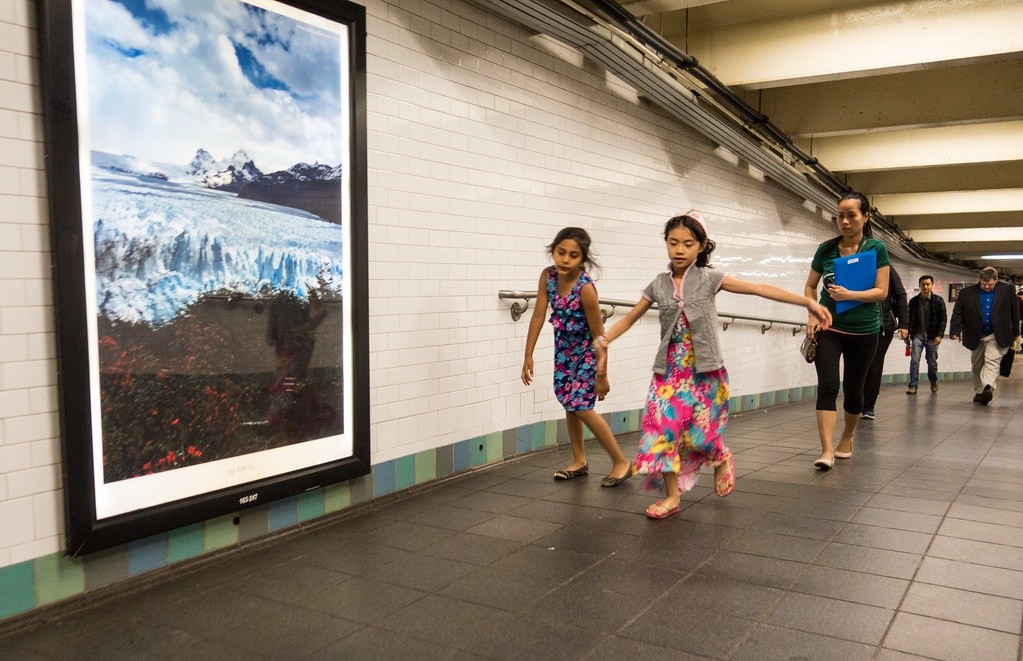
[800,328,821,363]
[999,348,1016,377]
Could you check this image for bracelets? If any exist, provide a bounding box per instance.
[592,335,610,349]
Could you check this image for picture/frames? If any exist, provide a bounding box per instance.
[37,1,374,558]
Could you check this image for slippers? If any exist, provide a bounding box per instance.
[646,499,680,519]
[713,455,736,497]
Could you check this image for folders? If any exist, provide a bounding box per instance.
[832,249,878,314]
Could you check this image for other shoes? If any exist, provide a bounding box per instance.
[834,437,853,458]
[814,457,835,470]
[931,382,938,393]
[554,461,590,480]
[600,464,632,487]
[973,384,993,406]
[906,389,917,394]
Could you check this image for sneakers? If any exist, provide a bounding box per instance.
[860,411,875,419]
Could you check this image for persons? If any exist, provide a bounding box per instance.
[859,264,910,420]
[1016,290,1023,355]
[805,193,891,470]
[521,226,635,488]
[591,208,833,519]
[904,274,948,395]
[949,267,1020,407]
